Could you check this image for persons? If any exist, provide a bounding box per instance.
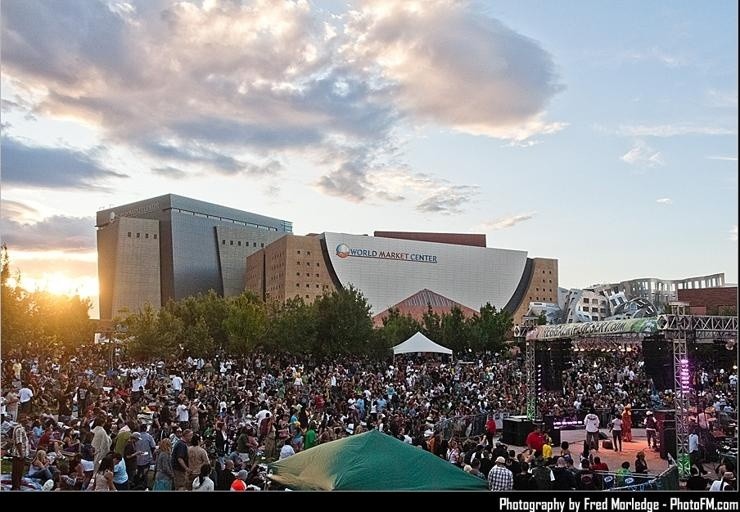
[1,339,739,492]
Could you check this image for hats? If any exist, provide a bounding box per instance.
[646,411,653,415]
[10,384,17,388]
[134,431,143,441]
[495,456,506,464]
[723,471,737,481]
